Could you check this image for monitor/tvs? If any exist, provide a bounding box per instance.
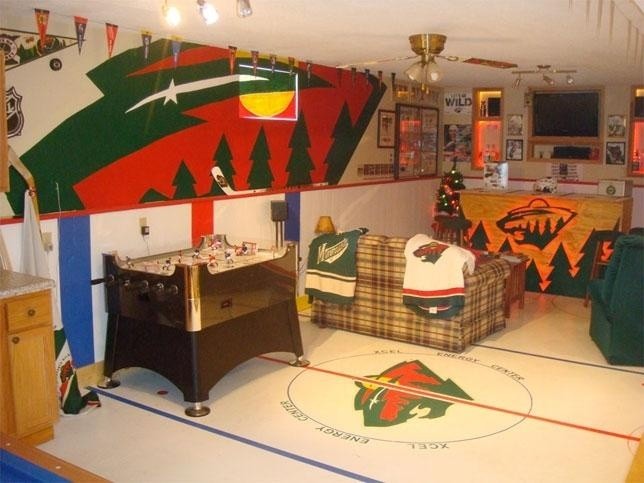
[533,92,599,137]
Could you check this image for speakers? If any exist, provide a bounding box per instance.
[488,97,500,116]
[271,200,288,222]
[634,95,644,117]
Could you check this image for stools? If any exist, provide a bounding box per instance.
[584,230,624,307]
[443,218,472,248]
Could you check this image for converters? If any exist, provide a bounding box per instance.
[142,226,150,235]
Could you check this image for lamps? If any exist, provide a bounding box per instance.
[160,0,253,28]
[512,64,577,87]
[406,59,445,93]
[314,215,337,234]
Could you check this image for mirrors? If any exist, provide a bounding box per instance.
[396,103,439,181]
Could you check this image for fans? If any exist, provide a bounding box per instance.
[335,33,518,94]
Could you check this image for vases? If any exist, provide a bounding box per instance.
[480,100,487,119]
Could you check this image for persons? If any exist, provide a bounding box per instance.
[125,239,249,273]
[444,125,466,153]
[509,143,516,159]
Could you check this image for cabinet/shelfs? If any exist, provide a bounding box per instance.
[0,291,57,446]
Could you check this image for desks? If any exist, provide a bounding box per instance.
[453,189,633,299]
[465,252,530,319]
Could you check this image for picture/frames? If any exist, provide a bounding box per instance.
[606,141,626,166]
[607,113,627,138]
[506,113,523,136]
[506,139,523,161]
[377,109,397,149]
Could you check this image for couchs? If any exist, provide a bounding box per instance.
[587,234,644,368]
[305,228,510,354]
